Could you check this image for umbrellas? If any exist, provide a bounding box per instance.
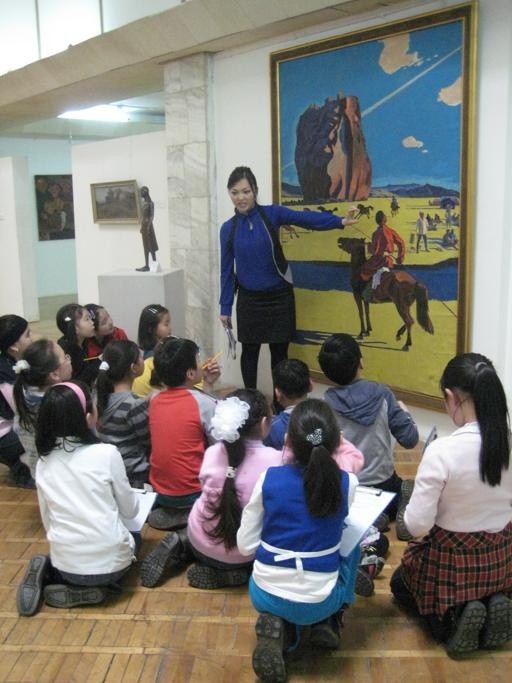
[443,591,510,659]
[12,508,252,614]
[247,606,340,683]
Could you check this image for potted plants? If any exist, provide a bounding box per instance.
[89,178,144,226]
[265,0,481,415]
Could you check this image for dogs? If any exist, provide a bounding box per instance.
[390,202,400,217]
[354,203,374,219]
[337,236,435,351]
[317,206,338,215]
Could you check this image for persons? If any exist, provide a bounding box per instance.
[220,166,361,414]
[2,315,36,490]
[83,304,128,362]
[280,429,390,595]
[389,353,511,656]
[263,358,312,449]
[93,340,152,489]
[353,210,405,282]
[140,388,284,590]
[415,203,458,252]
[12,338,98,486]
[137,186,157,270]
[132,304,204,400]
[237,398,362,680]
[144,336,222,529]
[17,379,140,616]
[318,333,417,532]
